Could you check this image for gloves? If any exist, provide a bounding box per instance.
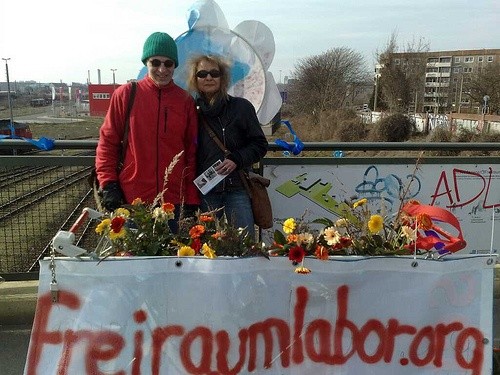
[101,182,123,212]
[183,204,198,221]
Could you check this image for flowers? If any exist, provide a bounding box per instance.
[92,198,432,275]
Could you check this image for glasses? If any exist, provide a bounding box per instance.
[195,70,221,79]
[148,59,175,69]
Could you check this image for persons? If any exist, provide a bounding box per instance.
[95,32,198,245]
[186,54,269,244]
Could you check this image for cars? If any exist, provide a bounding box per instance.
[0,119,33,154]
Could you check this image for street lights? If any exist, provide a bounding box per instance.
[1,57,18,155]
[110,68,117,92]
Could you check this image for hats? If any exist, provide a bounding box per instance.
[142,32,179,69]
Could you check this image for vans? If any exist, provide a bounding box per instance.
[30,99,49,108]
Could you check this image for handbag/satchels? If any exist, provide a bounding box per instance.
[88,83,137,190]
[244,170,273,230]
